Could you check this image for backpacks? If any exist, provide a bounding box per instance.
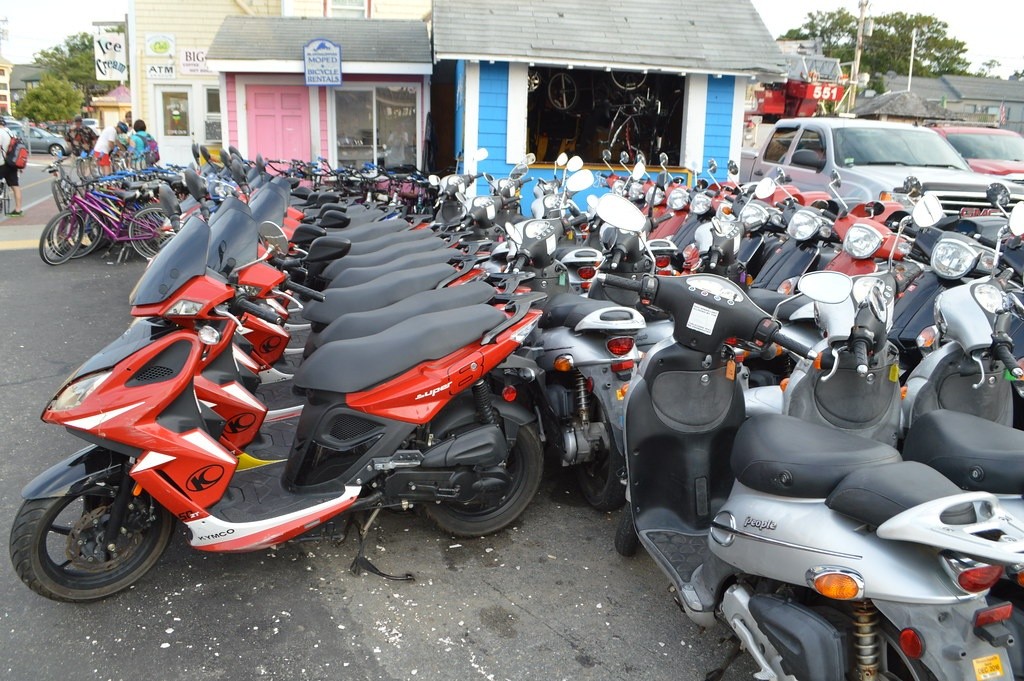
[1,129,28,171]
[135,133,160,165]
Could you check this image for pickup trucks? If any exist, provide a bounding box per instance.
[739,117,1024,219]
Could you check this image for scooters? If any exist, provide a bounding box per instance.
[10,146,1024,681]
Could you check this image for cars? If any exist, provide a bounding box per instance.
[7,125,73,157]
[930,126,1024,186]
[82,119,101,137]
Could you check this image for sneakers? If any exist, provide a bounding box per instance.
[5,209,23,217]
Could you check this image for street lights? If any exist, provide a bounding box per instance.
[14,94,19,105]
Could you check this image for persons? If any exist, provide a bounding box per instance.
[0,116,24,217]
[66,109,154,175]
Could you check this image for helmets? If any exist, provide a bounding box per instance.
[117,121,128,134]
